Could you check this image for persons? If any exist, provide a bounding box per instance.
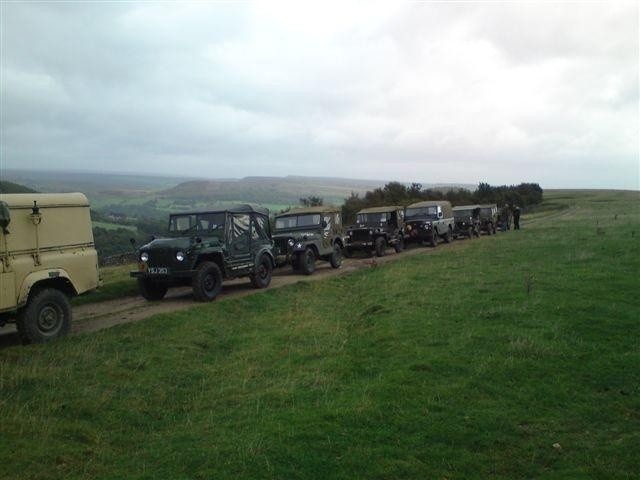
[512,205,522,230]
[505,204,512,231]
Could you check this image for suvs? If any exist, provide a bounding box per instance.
[129,202,278,304]
[344,205,408,259]
[405,199,513,250]
[268,206,346,275]
[0,191,103,343]
